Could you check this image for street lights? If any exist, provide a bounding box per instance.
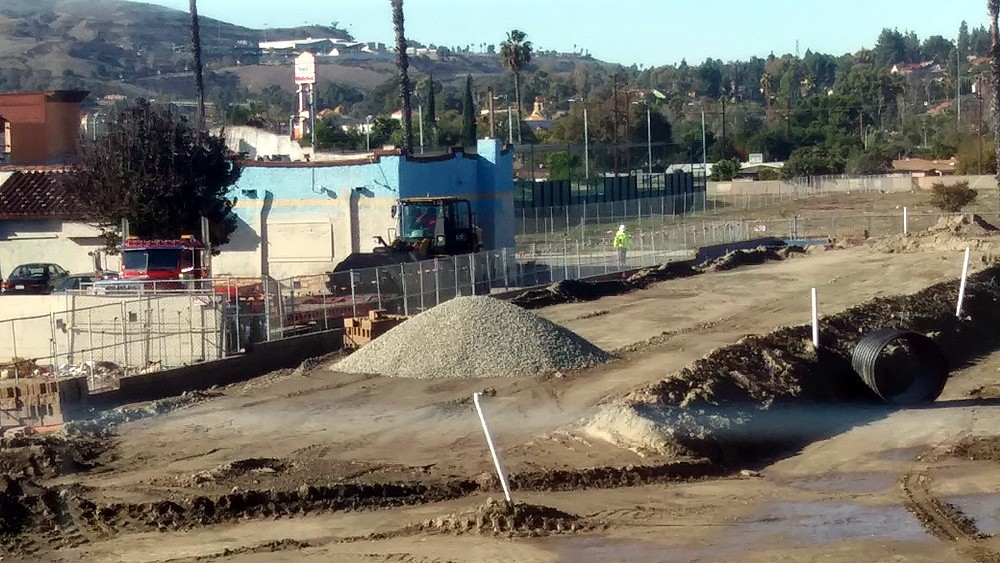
[567,98,588,179]
[631,101,652,175]
[687,102,707,190]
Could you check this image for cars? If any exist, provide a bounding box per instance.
[0,262,119,295]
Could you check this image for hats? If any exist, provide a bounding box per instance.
[618,225,627,231]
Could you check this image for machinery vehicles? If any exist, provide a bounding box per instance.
[324,193,497,296]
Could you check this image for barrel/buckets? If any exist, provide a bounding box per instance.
[536,265,550,284]
[518,264,537,285]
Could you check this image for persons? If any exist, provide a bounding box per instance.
[614,225,629,265]
[414,207,438,234]
